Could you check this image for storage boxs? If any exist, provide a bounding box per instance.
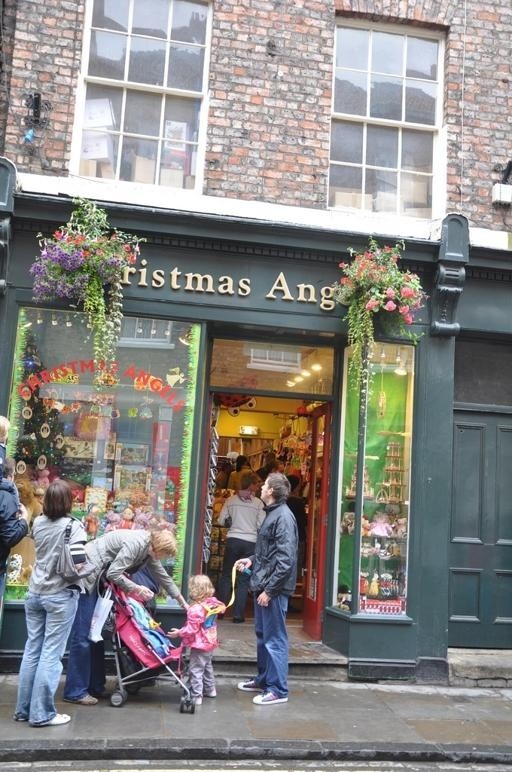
[328,165,432,218]
[80,97,197,189]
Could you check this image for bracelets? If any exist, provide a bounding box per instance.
[180,602,186,607]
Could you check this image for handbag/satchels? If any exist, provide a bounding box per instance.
[89,597,114,643]
[57,543,96,582]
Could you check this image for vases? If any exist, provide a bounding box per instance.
[371,305,403,342]
[101,281,114,309]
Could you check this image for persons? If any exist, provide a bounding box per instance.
[165,573,227,708]
[236,473,299,705]
[217,454,306,612]
[0,458,29,620]
[62,527,193,706]
[13,479,87,726]
[216,473,267,623]
[0,414,21,510]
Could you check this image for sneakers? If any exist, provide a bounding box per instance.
[237,680,264,692]
[89,690,113,698]
[253,692,288,704]
[14,714,71,727]
[63,694,98,705]
[180,686,216,705]
[233,617,245,622]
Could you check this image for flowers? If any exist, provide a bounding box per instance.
[28,197,148,390]
[331,234,430,393]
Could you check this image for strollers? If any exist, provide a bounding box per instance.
[94,573,198,713]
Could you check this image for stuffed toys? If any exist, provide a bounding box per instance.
[6,462,175,587]
[342,498,408,601]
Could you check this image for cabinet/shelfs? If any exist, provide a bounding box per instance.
[205,521,230,588]
